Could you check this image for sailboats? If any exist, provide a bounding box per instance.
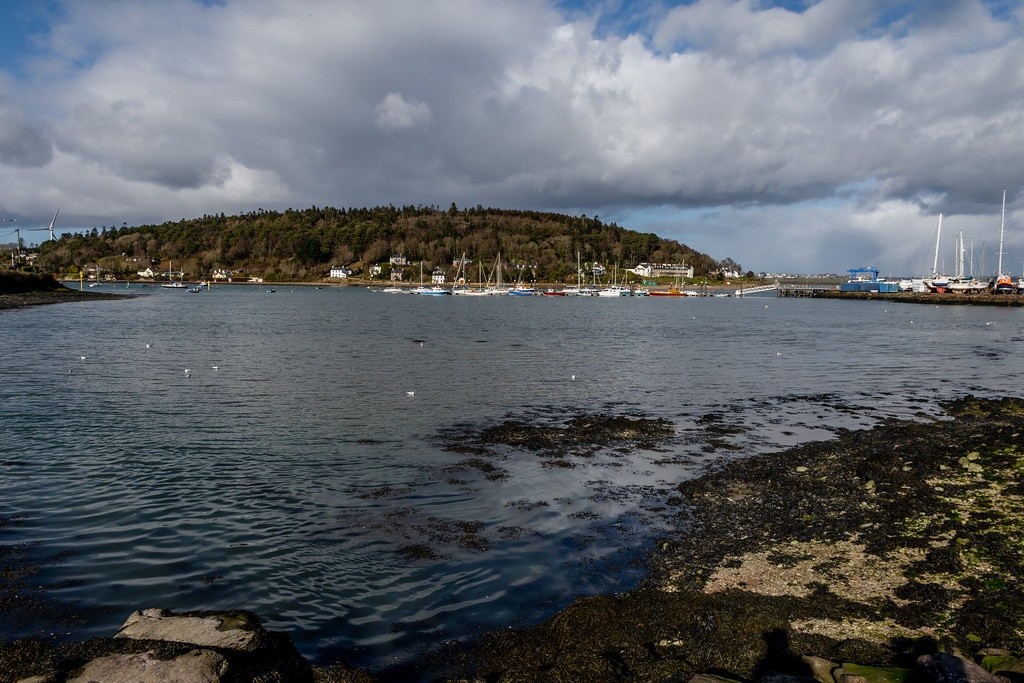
[161,261,187,288]
[367,250,734,297]
[895,188,1024,294]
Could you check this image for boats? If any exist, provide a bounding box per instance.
[267,289,275,293]
[90,282,102,287]
[316,287,323,290]
[186,289,199,293]
